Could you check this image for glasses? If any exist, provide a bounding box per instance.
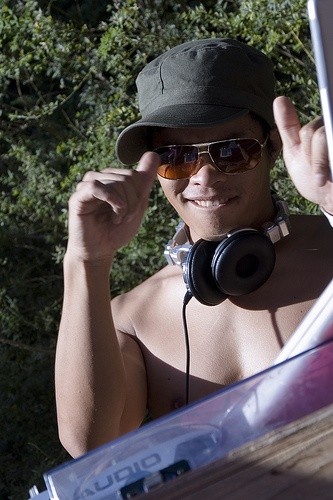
[149,135,269,181]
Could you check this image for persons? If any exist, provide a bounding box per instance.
[54,36,333,460]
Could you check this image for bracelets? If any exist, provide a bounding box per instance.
[320,207,333,228]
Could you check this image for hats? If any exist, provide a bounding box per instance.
[115,38,279,166]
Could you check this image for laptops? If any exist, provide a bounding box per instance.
[44,339,333,500]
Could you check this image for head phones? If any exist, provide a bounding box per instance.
[164,189,291,306]
